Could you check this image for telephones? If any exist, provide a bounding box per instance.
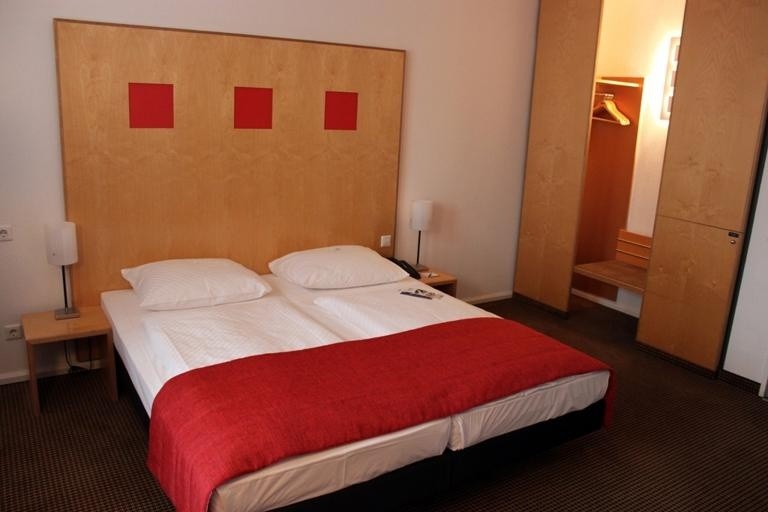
[389,257,420,279]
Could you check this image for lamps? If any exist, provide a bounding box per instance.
[408,200,434,273]
[45,220,82,320]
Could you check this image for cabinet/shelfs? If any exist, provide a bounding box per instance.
[630,0,767,381]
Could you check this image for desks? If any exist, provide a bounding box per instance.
[21,306,118,415]
[417,266,458,298]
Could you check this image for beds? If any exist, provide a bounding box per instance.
[98,271,618,512]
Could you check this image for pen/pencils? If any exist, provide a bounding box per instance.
[431,271,432,278]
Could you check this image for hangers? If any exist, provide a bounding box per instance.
[591,93,630,127]
